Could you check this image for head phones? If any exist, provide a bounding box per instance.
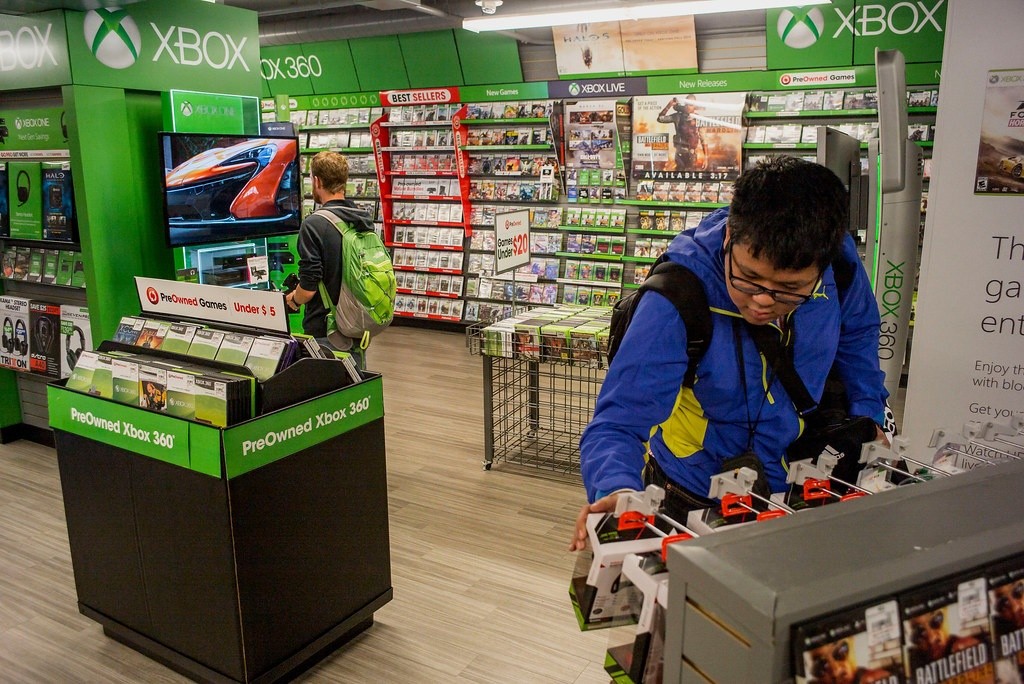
[14,318,28,356]
[2,317,14,350]
[35,316,54,355]
[65,326,85,371]
[61,110,67,138]
[17,170,31,202]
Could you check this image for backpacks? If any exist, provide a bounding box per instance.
[608,216,855,387]
[311,209,397,338]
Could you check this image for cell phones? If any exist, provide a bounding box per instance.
[722,451,770,508]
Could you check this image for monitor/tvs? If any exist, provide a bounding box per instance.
[157,132,302,248]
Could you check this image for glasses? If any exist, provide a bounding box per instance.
[728,235,824,306]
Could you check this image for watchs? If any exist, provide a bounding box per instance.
[291,294,301,307]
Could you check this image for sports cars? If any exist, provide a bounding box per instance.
[165,139,300,228]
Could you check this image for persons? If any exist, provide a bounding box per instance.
[570,154,890,550]
[657,95,708,172]
[286,151,374,352]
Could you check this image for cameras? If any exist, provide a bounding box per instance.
[788,407,877,478]
[282,273,300,295]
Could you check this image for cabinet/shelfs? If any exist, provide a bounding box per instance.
[1,1,1024,683]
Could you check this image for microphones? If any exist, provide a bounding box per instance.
[62,140,68,143]
[0,348,13,353]
[17,201,27,207]
[10,350,23,356]
